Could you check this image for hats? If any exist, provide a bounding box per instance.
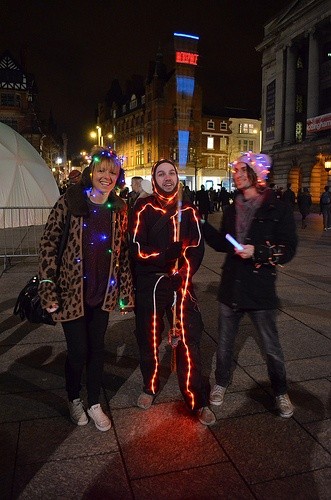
[238,152,273,186]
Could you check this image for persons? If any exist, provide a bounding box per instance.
[125,160,217,426]
[58,169,331,235]
[197,153,298,418]
[14,151,135,432]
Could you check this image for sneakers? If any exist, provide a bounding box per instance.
[67,398,90,426]
[275,392,295,419]
[209,383,229,406]
[86,403,112,432]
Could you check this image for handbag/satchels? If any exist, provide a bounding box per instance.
[13,274,58,326]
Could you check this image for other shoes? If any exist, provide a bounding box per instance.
[136,392,154,410]
[197,406,216,425]
